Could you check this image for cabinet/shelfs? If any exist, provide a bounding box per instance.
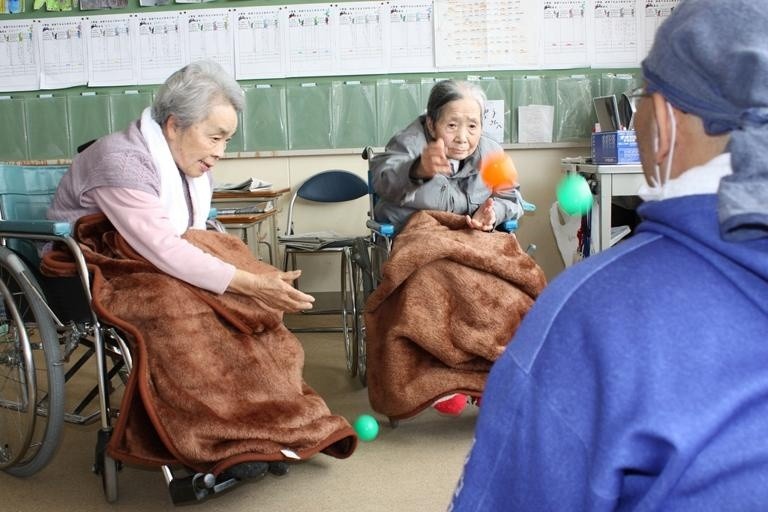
[562,159,655,270]
[206,181,292,265]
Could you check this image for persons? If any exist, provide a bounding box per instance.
[20,57,315,482]
[366,76,527,422]
[441,0,767,512]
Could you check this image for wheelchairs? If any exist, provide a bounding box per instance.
[337,147,540,427]
[0,140,299,509]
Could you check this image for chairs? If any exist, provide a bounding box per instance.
[279,170,373,332]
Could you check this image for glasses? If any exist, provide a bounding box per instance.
[625,87,652,113]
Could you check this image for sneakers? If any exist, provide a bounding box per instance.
[227,460,270,484]
